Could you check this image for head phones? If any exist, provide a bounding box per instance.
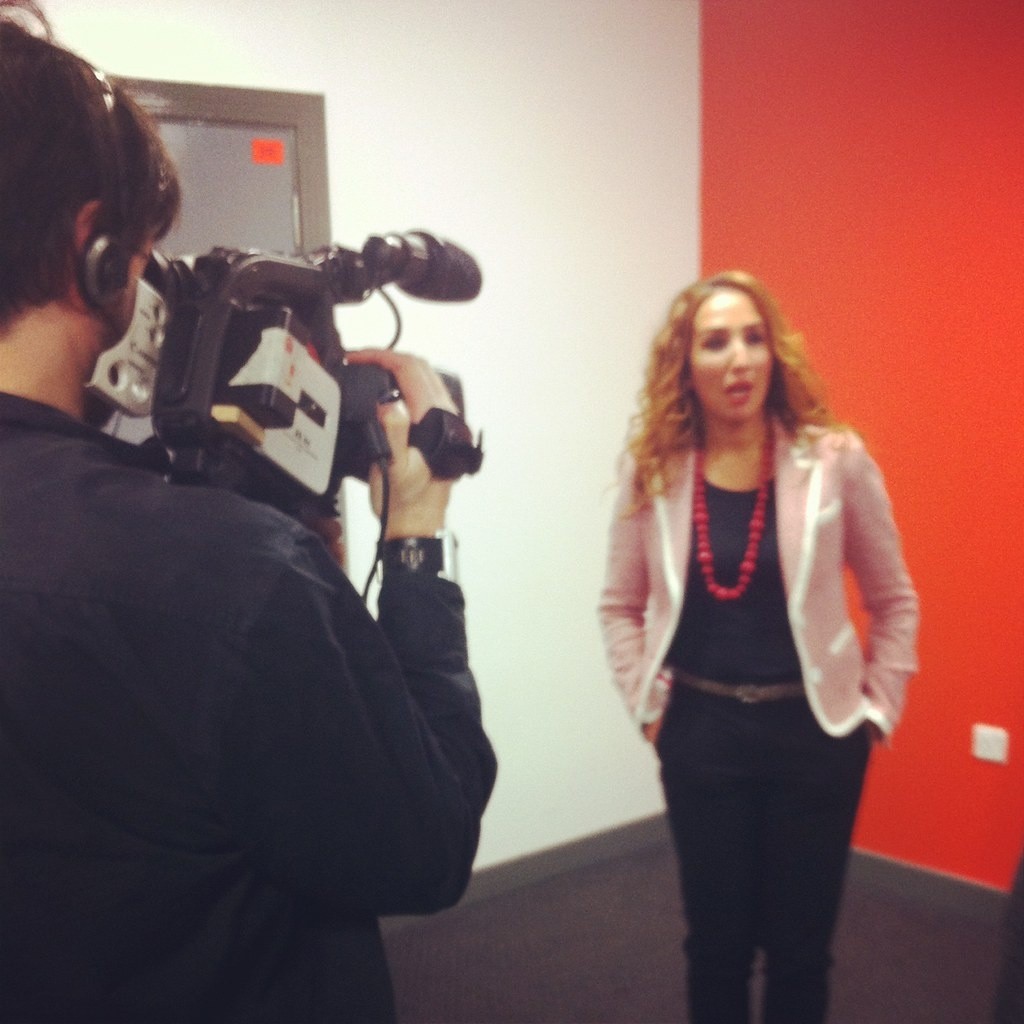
[72,56,135,311]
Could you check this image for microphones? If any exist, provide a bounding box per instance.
[371,228,481,302]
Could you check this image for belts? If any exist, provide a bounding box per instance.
[678,672,806,703]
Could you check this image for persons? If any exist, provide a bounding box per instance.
[598,271,920,1024]
[0,17,497,1024]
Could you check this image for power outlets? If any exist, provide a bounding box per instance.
[972,722,1006,762]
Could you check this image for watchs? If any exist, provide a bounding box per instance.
[379,531,458,575]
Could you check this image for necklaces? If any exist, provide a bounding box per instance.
[693,418,774,601]
